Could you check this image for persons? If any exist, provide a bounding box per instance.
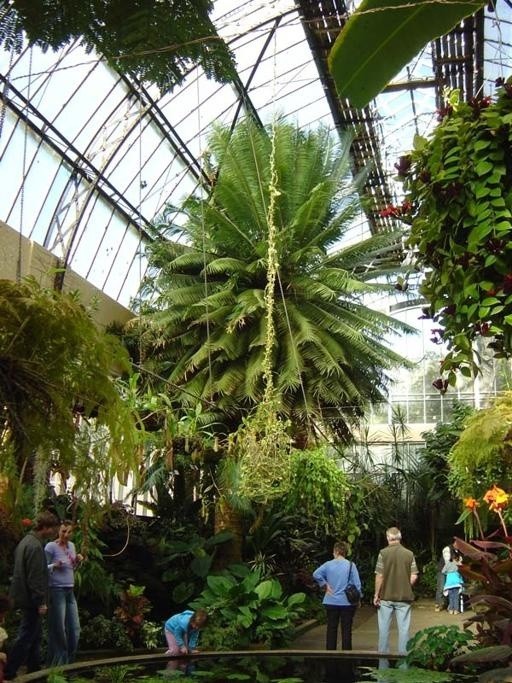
[435,542,463,613]
[4,516,49,679]
[313,541,363,650]
[45,520,84,668]
[164,609,209,655]
[442,549,463,614]
[377,658,408,683]
[373,527,420,653]
[165,659,197,677]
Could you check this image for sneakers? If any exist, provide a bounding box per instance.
[435,602,460,615]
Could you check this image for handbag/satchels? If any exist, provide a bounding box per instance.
[344,584,360,604]
